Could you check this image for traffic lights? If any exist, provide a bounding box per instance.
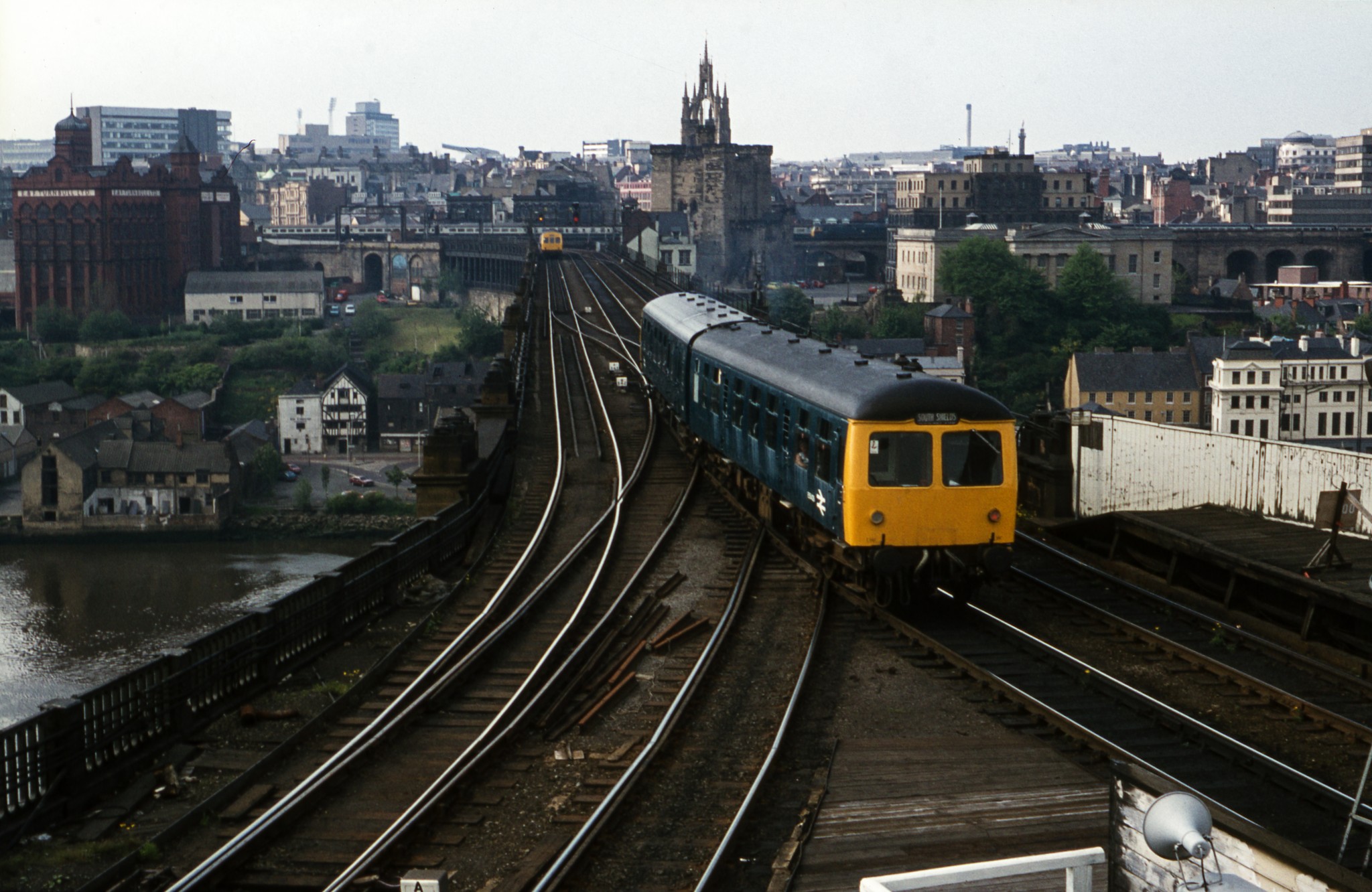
[574,212,579,222]
[539,213,543,222]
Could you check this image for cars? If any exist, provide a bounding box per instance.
[349,475,374,488]
[334,290,348,302]
[376,294,388,305]
[361,490,385,501]
[329,305,339,317]
[340,490,363,501]
[766,279,824,291]
[282,470,297,482]
[868,285,883,293]
[286,463,302,474]
[345,304,355,315]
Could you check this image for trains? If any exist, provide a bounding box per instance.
[639,290,1022,610]
[538,228,563,255]
